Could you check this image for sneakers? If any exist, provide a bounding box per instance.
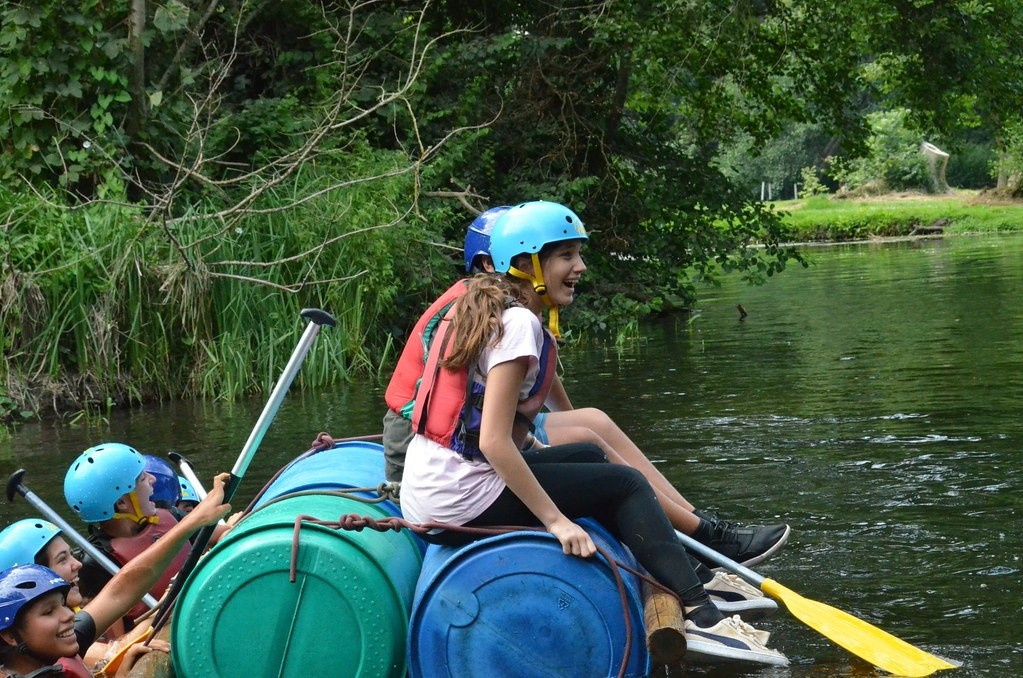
[702,571,778,621]
[684,615,788,666]
[685,517,790,573]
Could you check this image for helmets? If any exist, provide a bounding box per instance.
[0,519,62,573]
[464,206,518,272]
[0,564,71,630]
[64,443,146,523]
[490,200,588,273]
[178,476,200,503]
[142,455,181,501]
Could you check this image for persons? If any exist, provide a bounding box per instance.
[382,199,792,670]
[0,442,246,677]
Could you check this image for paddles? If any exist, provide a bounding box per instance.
[7,465,161,609]
[675,529,965,677]
[95,306,340,678]
